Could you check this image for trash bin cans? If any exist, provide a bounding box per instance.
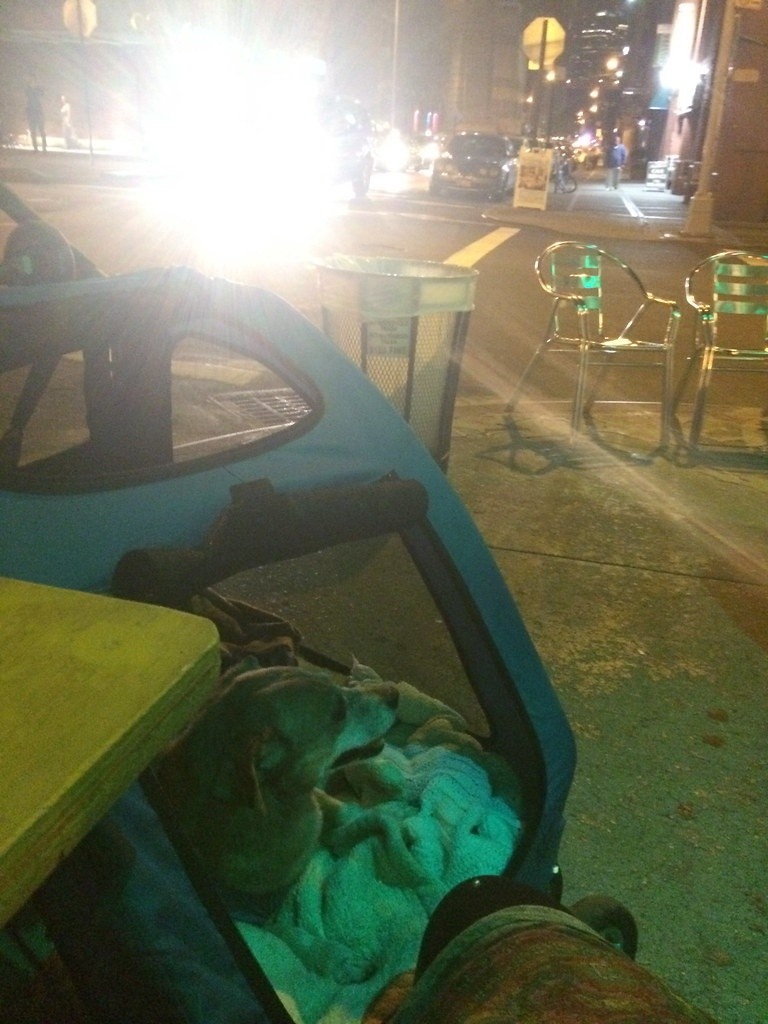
[318,252,479,476]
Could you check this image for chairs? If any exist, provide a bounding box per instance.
[504,240,680,451]
[673,250,768,461]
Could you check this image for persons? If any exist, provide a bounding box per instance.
[59,97,74,145]
[605,137,627,190]
[26,79,47,152]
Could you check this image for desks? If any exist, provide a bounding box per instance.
[0,575,220,928]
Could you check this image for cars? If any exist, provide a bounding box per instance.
[427,128,574,205]
